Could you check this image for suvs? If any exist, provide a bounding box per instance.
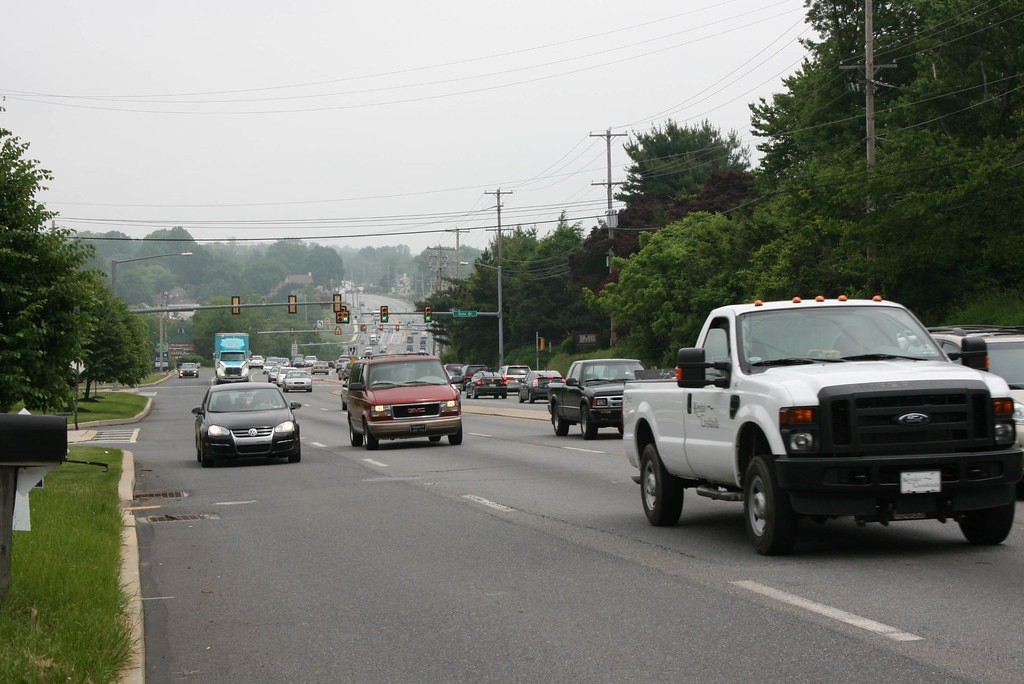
[497,364,532,391]
[897,323,1023,459]
[249,355,265,369]
[443,363,465,390]
[457,363,490,391]
[347,352,463,450]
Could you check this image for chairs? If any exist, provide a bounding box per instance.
[251,392,270,410]
[577,374,597,381]
[214,394,234,413]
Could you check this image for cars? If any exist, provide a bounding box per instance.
[263,354,318,383]
[465,371,508,399]
[177,361,202,378]
[311,361,330,375]
[191,381,302,467]
[518,370,566,404]
[334,307,429,410]
[283,370,314,392]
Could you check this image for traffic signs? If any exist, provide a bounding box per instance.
[454,311,478,318]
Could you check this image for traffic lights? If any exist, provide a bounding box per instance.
[335,306,349,323]
[380,306,388,323]
[424,306,432,322]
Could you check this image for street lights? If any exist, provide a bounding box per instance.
[460,261,503,369]
[112,252,193,293]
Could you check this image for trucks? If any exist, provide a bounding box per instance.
[212,333,253,384]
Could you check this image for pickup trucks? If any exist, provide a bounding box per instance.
[547,358,649,440]
[621,294,1023,557]
